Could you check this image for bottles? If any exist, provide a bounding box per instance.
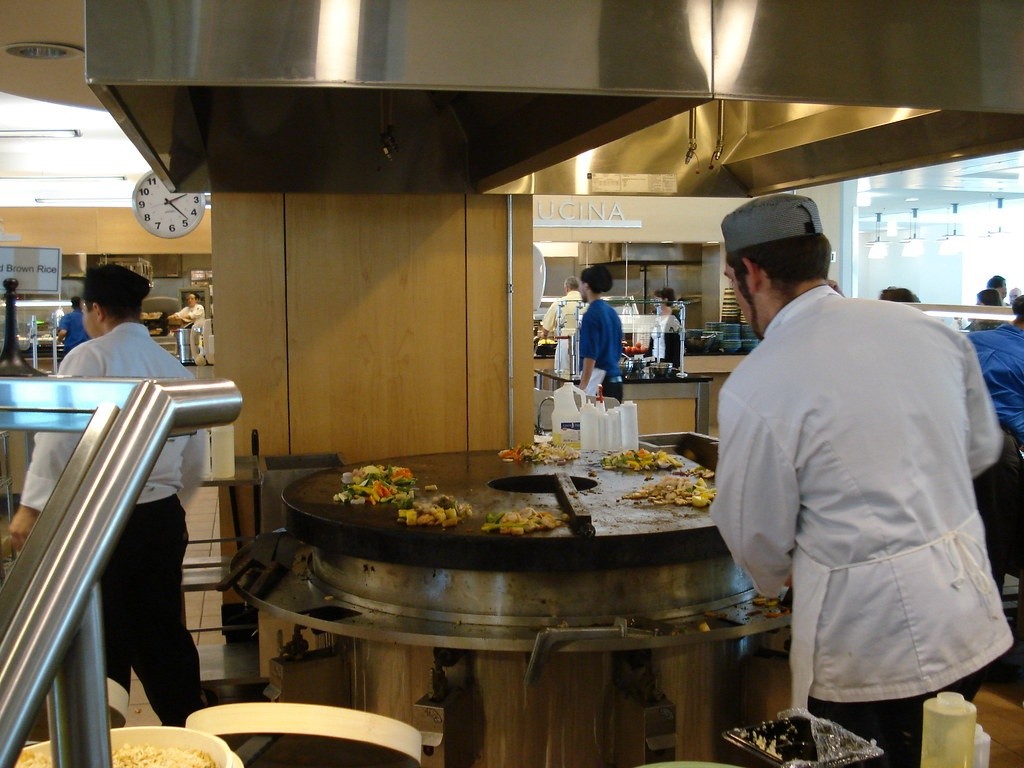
[920,692,977,768]
[974,723,991,768]
[551,381,639,452]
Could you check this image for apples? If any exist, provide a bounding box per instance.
[625,342,646,353]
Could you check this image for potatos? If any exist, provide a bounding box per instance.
[692,477,709,507]
[358,486,457,525]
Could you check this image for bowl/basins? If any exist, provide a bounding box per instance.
[11,725,246,768]
[617,358,673,379]
[685,287,761,354]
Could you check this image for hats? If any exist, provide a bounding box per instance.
[720,194,823,253]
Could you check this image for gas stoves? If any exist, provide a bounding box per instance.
[536,348,555,356]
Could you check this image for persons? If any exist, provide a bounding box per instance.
[965,275,1024,442]
[709,195,1015,768]
[9,264,210,726]
[57,297,90,354]
[879,287,919,302]
[579,266,623,404]
[167,293,205,322]
[540,277,590,368]
[646,288,680,365]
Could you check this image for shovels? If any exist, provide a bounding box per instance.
[214,532,299,596]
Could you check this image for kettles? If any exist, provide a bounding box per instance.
[172,328,196,366]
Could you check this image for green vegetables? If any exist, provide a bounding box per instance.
[481,512,528,532]
[601,449,641,467]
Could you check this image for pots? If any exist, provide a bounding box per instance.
[535,335,558,351]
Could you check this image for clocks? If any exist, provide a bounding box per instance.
[131,169,206,239]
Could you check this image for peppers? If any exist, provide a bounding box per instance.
[341,463,460,512]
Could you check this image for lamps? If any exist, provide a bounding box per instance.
[936,207,955,255]
[977,198,1013,251]
[867,213,891,260]
[904,207,930,254]
[899,212,918,257]
[942,203,966,251]
